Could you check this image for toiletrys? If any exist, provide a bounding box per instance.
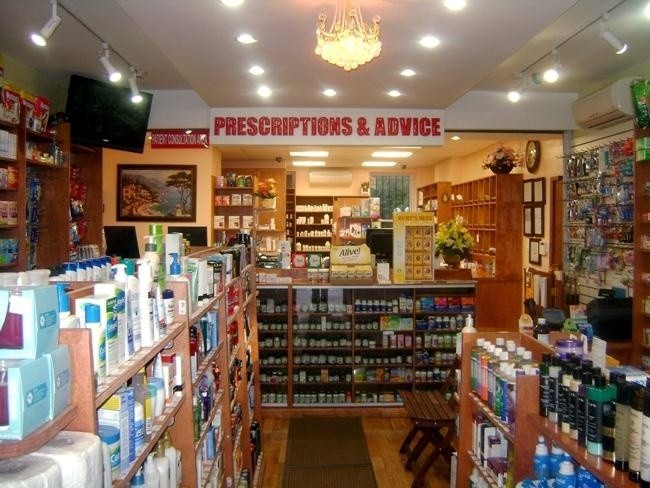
[471,300,649,488]
[185,251,224,486]
[59,231,186,487]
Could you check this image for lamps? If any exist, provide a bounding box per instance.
[31,0,144,104]
[315,0,382,71]
[506,0,629,102]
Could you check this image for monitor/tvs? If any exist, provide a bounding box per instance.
[64,74,154,155]
[366,228,394,268]
[103,225,140,258]
[168,226,208,246]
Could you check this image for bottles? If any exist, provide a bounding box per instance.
[565,279,580,309]
[296,213,332,224]
[355,295,412,364]
[462,314,478,333]
[293,303,352,383]
[532,317,551,346]
[468,468,479,488]
[261,390,378,404]
[296,240,330,252]
[516,436,605,488]
[469,337,539,432]
[257,304,289,384]
[304,204,333,212]
[415,315,464,383]
[539,352,650,488]
[297,228,332,237]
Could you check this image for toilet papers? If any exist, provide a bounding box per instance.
[1,430,103,488]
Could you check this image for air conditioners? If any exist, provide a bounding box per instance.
[571,77,644,131]
